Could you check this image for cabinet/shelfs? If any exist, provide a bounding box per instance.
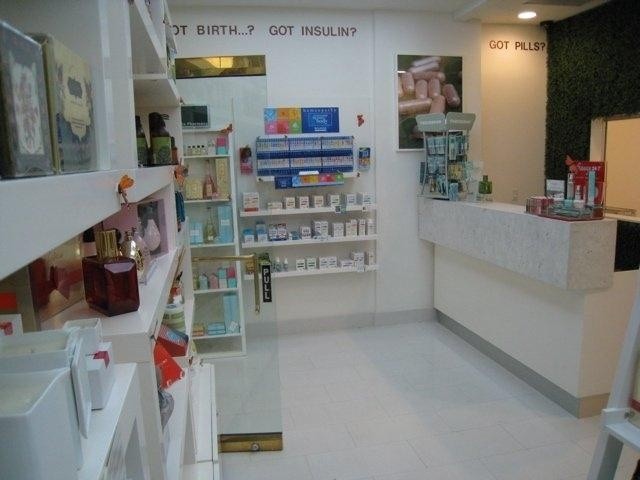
[237,201,379,280]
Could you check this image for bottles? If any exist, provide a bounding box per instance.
[204,205,216,243]
[183,144,206,155]
[477,173,492,201]
[565,173,582,208]
[202,159,216,199]
[275,255,289,273]
[83,228,140,316]
